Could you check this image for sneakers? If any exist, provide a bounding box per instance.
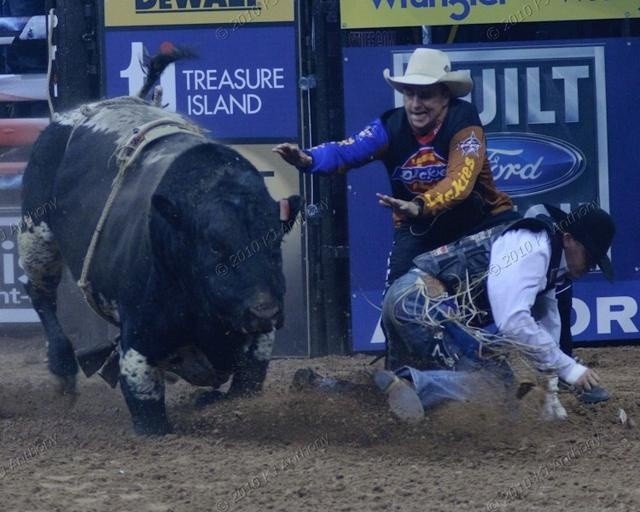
[557,373,610,404]
[372,369,426,426]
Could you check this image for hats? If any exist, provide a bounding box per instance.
[383,47,474,98]
[543,199,617,285]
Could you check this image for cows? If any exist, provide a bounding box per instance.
[16,45,305,440]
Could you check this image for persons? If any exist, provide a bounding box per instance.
[273,47,573,395]
[289,204,615,426]
